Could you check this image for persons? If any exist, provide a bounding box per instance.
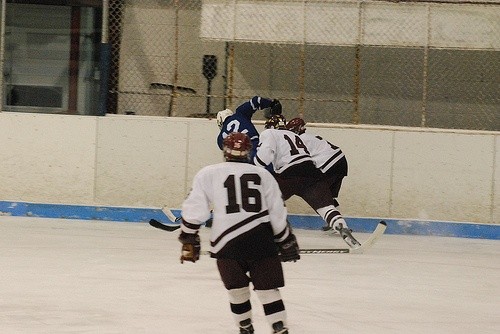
[179,132,299,334]
[216,96,362,248]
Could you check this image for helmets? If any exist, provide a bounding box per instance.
[286,118,306,134]
[217,108,234,130]
[265,114,286,129]
[223,132,253,161]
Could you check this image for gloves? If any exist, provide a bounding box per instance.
[279,233,301,263]
[270,98,282,114]
[178,232,201,262]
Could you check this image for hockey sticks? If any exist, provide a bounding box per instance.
[149,218,181,232]
[181,220,388,258]
[161,203,182,223]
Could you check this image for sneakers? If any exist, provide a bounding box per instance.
[321,224,337,232]
[335,221,362,248]
